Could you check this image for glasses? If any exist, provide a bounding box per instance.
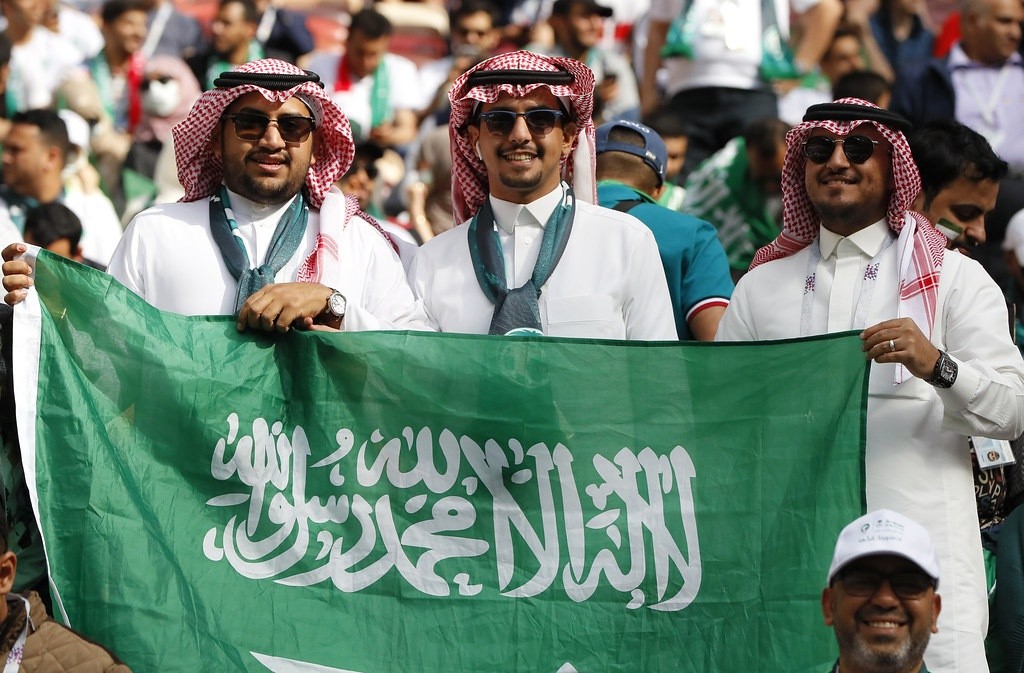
[801,133,892,164]
[138,75,170,92]
[223,112,317,143]
[475,108,567,135]
[832,569,935,599]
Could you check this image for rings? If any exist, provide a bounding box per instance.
[889,340,895,351]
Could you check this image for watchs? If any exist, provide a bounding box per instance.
[923,349,959,389]
[319,287,347,324]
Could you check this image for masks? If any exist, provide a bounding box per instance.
[142,80,178,114]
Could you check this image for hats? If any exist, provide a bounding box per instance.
[554,0,614,19]
[594,119,667,188]
[829,508,940,591]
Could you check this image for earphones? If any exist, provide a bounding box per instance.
[476,138,483,160]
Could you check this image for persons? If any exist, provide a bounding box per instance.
[407,49,694,476]
[712,98,1024,673]
[0,498,135,673]
[0,58,439,512]
[822,509,942,673]
[987,451,1003,462]
[0,0,1024,673]
